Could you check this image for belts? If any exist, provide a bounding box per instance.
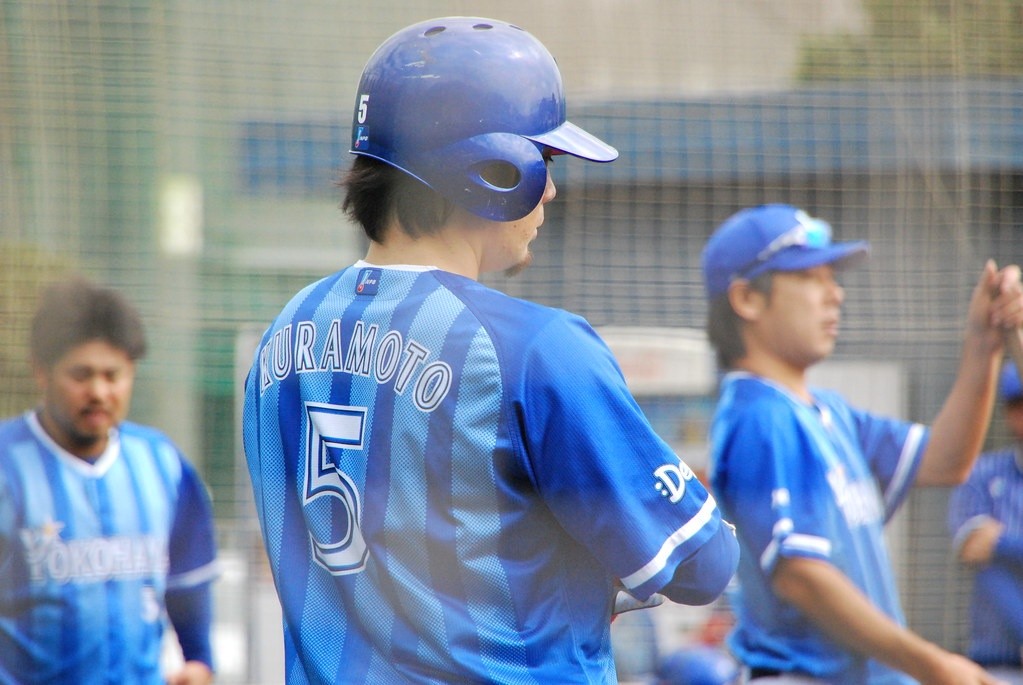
[748,664,781,679]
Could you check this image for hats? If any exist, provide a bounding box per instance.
[705,201,871,293]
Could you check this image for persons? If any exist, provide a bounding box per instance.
[651,457,756,685]
[0,282,222,685]
[245,21,742,685]
[956,362,1023,684]
[710,198,1023,685]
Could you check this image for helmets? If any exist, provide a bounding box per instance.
[348,16,620,224]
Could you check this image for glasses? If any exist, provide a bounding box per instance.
[740,216,834,272]
